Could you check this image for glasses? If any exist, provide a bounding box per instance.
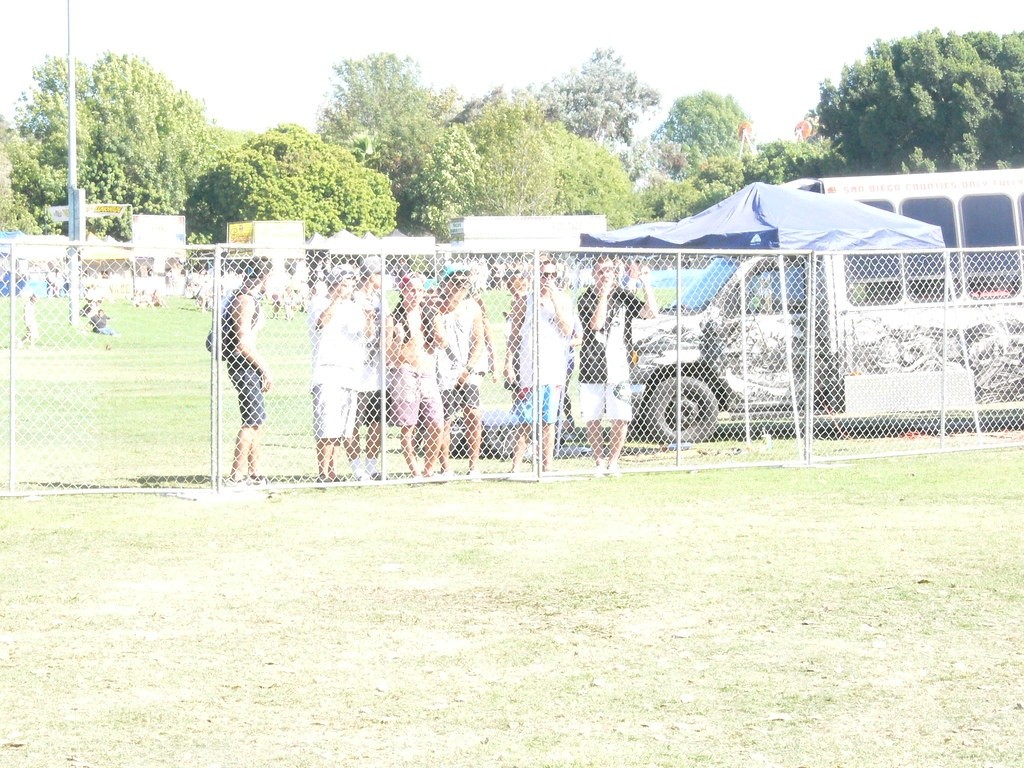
[541,272,558,277]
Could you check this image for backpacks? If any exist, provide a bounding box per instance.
[206,293,259,360]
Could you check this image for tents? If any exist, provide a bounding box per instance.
[578,182,981,457]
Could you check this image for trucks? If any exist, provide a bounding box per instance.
[631,167,1024,444]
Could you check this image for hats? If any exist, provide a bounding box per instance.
[326,264,355,283]
[243,256,271,287]
[437,265,470,284]
[400,273,426,288]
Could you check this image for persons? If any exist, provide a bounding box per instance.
[23,293,38,345]
[310,264,372,483]
[575,255,657,478]
[219,256,272,488]
[387,272,450,486]
[44,262,308,321]
[346,266,396,490]
[88,310,120,335]
[505,260,574,473]
[416,257,642,484]
